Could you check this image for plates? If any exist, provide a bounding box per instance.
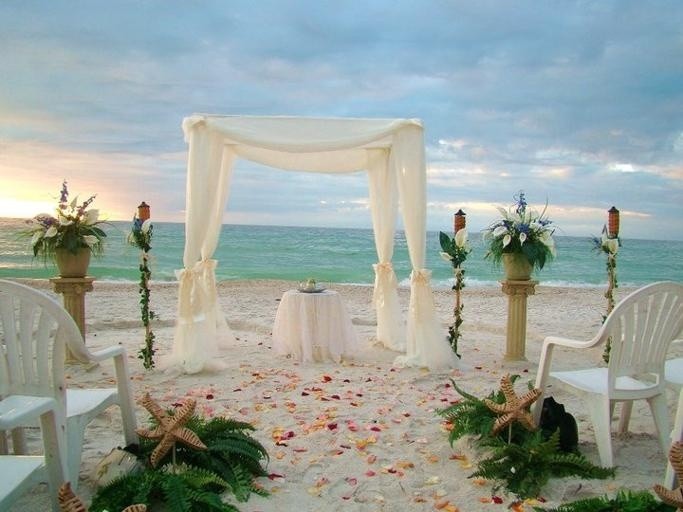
[296,285,326,293]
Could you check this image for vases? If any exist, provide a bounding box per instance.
[54,244,92,277]
[502,251,532,282]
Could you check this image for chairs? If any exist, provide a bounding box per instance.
[524,279,683,498]
[0,277,140,512]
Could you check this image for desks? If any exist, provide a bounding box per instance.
[272,291,362,365]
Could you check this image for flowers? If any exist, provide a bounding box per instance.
[15,177,119,270]
[480,190,561,273]
